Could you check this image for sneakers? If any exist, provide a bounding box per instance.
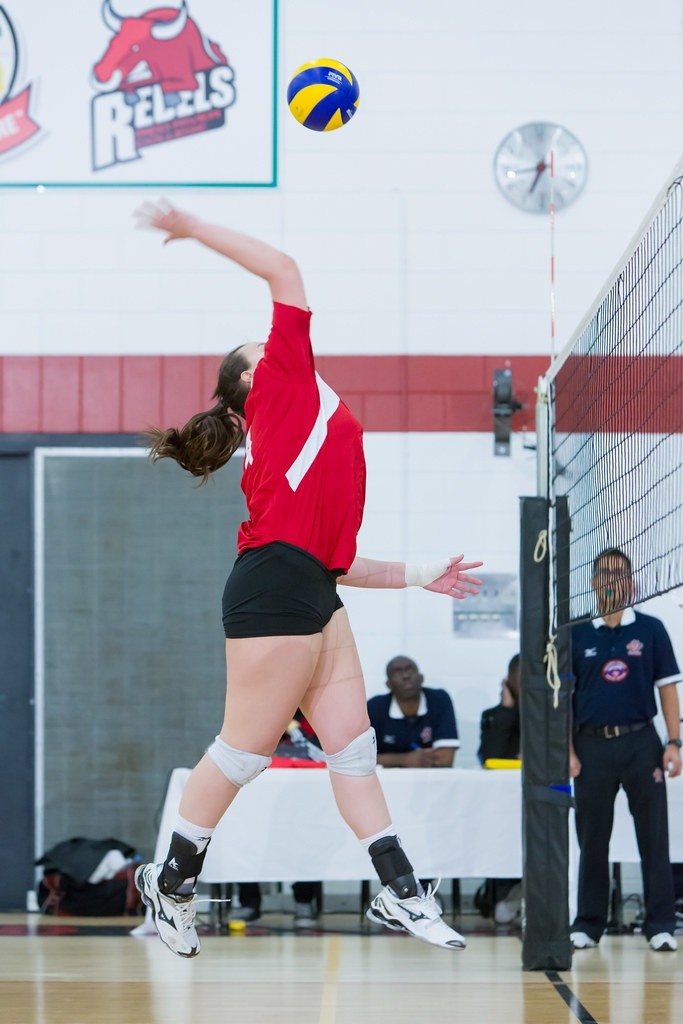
[221,904,264,927]
[569,931,598,949]
[134,862,201,958]
[293,902,316,929]
[650,931,678,951]
[366,877,467,951]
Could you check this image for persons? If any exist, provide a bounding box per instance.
[136,197,467,961]
[368,656,460,767]
[482,548,682,951]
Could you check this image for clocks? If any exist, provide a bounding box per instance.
[493,122,586,215]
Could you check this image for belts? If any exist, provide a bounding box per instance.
[575,719,655,739]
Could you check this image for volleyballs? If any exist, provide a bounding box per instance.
[286,57,361,133]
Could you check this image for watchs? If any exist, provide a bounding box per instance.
[668,739,682,747]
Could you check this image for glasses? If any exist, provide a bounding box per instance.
[592,568,629,582]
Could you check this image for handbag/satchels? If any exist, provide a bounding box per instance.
[31,837,136,917]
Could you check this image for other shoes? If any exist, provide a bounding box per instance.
[494,883,523,923]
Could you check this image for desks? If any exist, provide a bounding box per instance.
[155,766,683,932]
